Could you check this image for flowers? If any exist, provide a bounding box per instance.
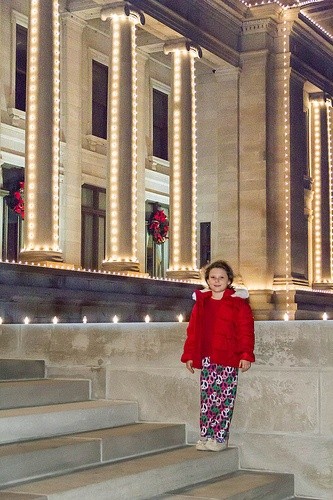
[4,182,25,219]
[150,209,169,246]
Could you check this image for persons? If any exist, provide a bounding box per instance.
[181,260,256,452]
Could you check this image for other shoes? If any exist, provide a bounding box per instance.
[205,438,228,451]
[194,438,210,450]
